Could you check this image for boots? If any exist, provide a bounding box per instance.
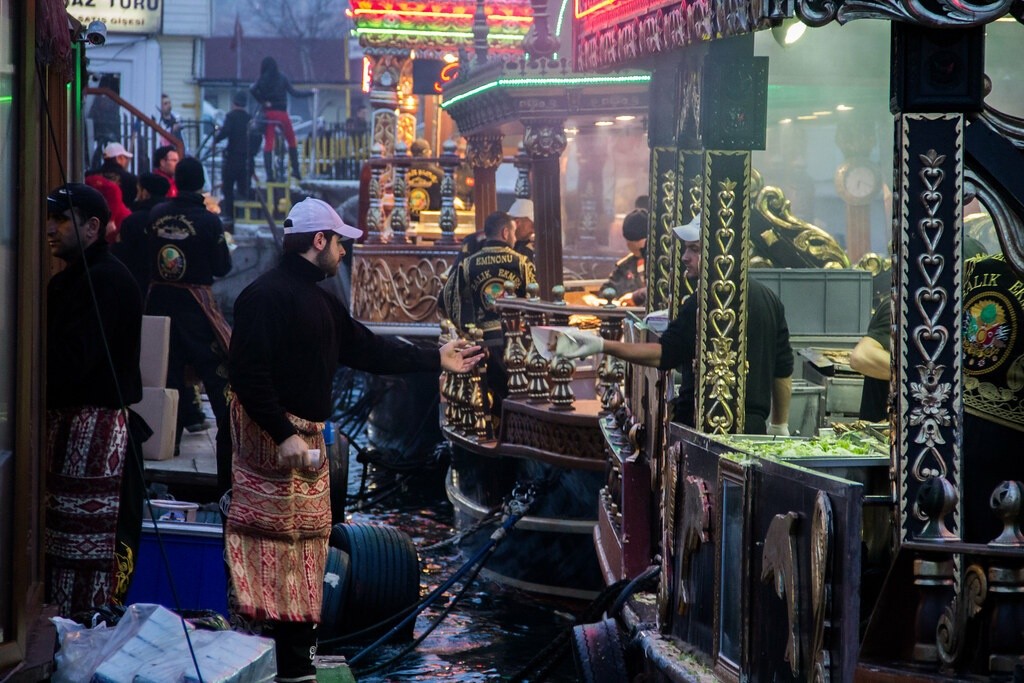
[288,147,301,180]
[264,150,276,181]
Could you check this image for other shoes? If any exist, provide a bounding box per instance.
[274,664,315,681]
[186,420,212,432]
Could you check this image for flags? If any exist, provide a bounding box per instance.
[230,20,243,50]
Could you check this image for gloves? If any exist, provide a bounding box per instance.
[562,329,602,359]
[766,421,788,435]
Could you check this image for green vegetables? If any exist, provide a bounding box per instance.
[708,431,874,465]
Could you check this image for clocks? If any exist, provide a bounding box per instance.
[836,159,883,207]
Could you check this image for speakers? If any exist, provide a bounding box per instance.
[890,20,986,114]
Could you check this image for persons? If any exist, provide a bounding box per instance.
[438,199,534,438]
[225,198,485,683]
[249,57,317,183]
[334,194,360,277]
[36,183,153,629]
[145,156,234,517]
[597,194,650,307]
[850,234,988,426]
[160,94,186,148]
[86,141,212,435]
[409,141,445,222]
[213,91,262,220]
[565,212,795,436]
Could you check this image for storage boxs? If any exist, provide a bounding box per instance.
[128,387,180,461]
[141,315,171,387]
[126,519,229,619]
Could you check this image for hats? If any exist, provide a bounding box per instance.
[46,181,109,224]
[672,211,703,242]
[284,196,363,238]
[232,90,247,107]
[507,198,534,221]
[175,156,204,191]
[103,142,133,160]
[623,208,649,240]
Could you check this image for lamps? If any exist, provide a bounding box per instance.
[772,12,809,48]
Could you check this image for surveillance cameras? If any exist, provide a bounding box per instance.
[87,22,106,45]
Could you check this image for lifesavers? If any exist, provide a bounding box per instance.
[320,522,421,640]
[570,618,631,683]
